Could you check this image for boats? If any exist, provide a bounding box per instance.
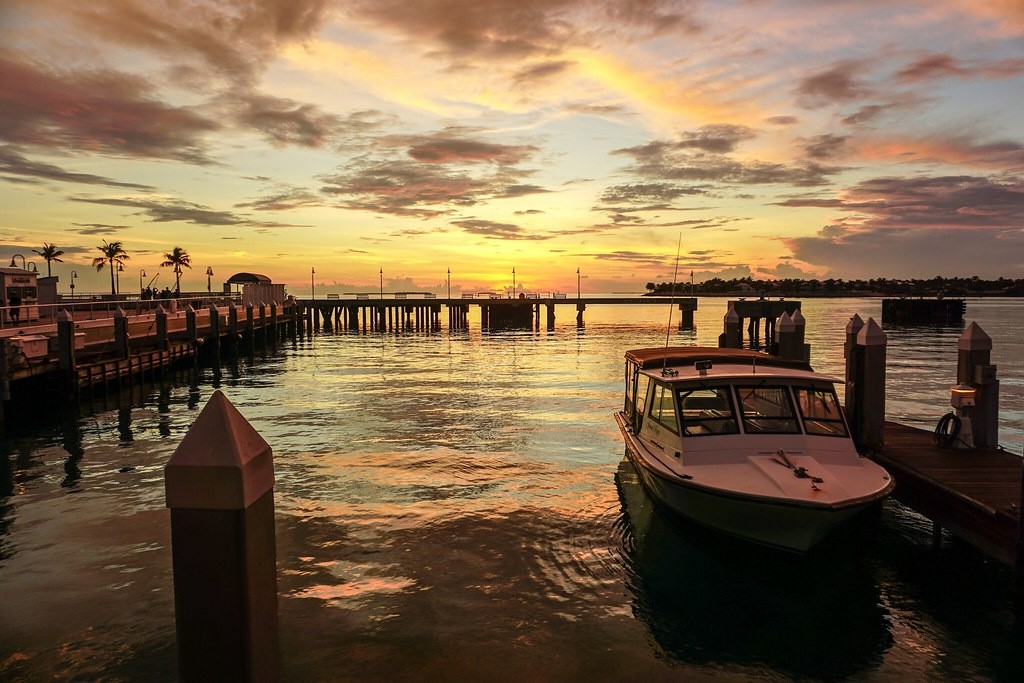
[612,231,899,558]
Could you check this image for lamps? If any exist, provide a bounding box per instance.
[951,382,977,408]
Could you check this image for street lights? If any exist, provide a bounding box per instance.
[206,266,213,292]
[69,270,78,303]
[116,261,125,301]
[311,266,316,300]
[174,265,182,293]
[576,267,581,299]
[512,267,516,299]
[379,267,383,299]
[139,269,146,300]
[690,270,693,298]
[447,267,451,299]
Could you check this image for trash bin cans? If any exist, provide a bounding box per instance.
[169,299,177,313]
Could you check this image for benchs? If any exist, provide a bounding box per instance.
[462,294,473,299]
[425,294,436,299]
[526,294,566,299]
[395,293,407,299]
[685,409,735,434]
[356,293,369,300]
[489,294,501,299]
[327,294,340,300]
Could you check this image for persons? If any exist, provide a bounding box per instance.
[141,287,180,309]
[9,291,21,326]
